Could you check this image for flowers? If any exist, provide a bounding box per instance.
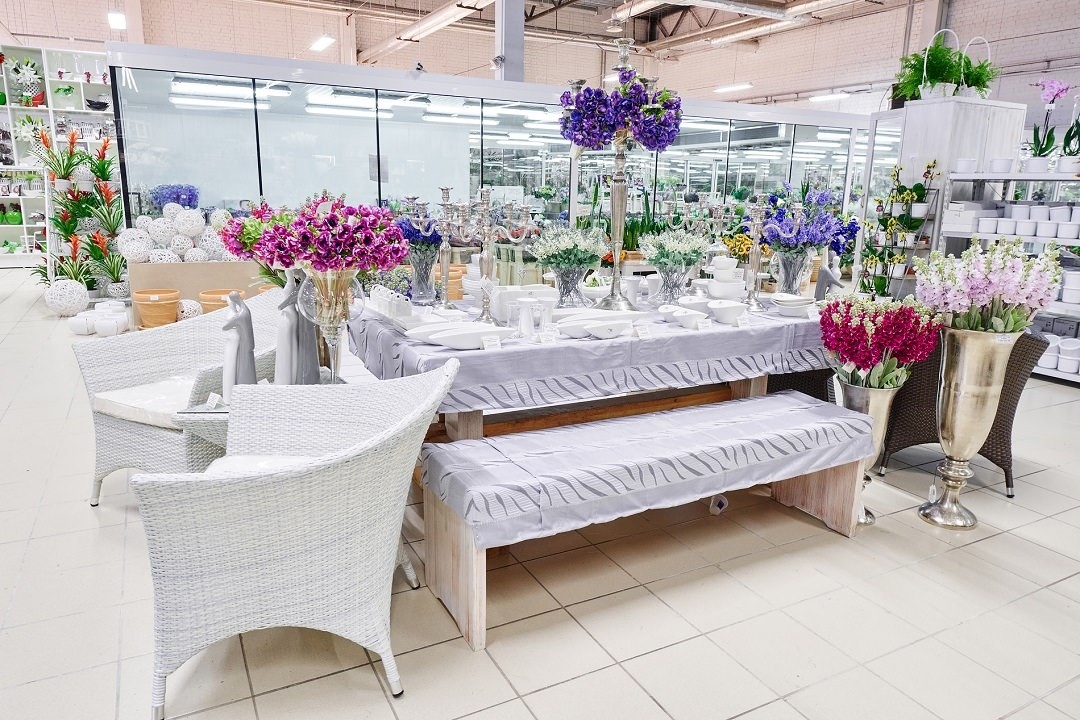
[0,52,128,286]
[224,69,1080,389]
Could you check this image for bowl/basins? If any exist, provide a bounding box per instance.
[657,256,817,328]
[978,206,1080,238]
[86,99,109,111]
[23,190,40,196]
[461,254,499,299]
[550,307,647,340]
[1030,323,1080,374]
[1052,267,1080,304]
[958,159,975,174]
[991,158,1013,173]
[583,286,610,299]
[392,315,513,350]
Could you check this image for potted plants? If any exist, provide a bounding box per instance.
[892,30,1001,102]
[17,175,42,198]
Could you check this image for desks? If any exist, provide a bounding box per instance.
[347,292,838,559]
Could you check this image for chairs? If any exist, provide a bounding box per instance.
[878,313,1049,498]
[71,285,283,507]
[130,358,459,720]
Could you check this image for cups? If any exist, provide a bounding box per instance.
[506,301,543,332]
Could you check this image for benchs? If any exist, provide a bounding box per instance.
[420,389,873,653]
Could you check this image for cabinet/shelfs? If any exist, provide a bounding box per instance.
[853,188,939,302]
[936,172,1080,382]
[0,45,132,269]
[44,164,132,305]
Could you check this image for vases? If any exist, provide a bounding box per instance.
[0,84,278,336]
[297,244,905,527]
[855,157,1079,531]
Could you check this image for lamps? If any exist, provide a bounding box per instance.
[489,54,505,69]
